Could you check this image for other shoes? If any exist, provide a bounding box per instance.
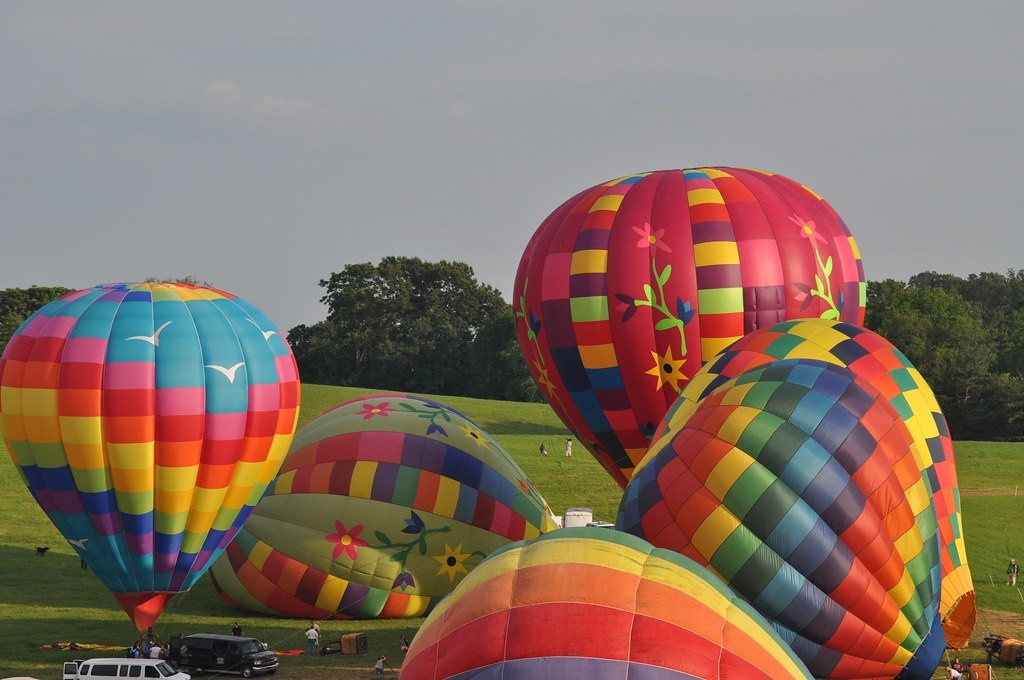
[1006,582,1009,585]
[85,568,87,570]
[377,673,378,675]
[1012,582,1016,585]
[566,457,568,458]
[380,674,381,675]
[81,567,83,569]
[569,457,571,458]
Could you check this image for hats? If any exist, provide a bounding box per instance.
[150,641,154,646]
[1011,559,1014,561]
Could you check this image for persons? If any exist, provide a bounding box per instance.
[303,619,322,656]
[565,438,572,457]
[375,655,386,676]
[127,638,169,662]
[231,622,244,636]
[945,656,972,680]
[399,633,409,657]
[1006,559,1017,586]
[539,442,547,457]
[259,639,268,651]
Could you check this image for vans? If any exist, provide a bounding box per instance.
[64,657,191,680]
[171,633,279,678]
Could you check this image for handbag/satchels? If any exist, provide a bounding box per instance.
[401,646,408,650]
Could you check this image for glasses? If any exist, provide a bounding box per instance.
[1010,561,1012,562]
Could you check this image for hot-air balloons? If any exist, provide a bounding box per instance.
[2,282,302,661]
[396,527,815,679]
[614,317,1022,677]
[510,164,867,490]
[207,395,568,619]
